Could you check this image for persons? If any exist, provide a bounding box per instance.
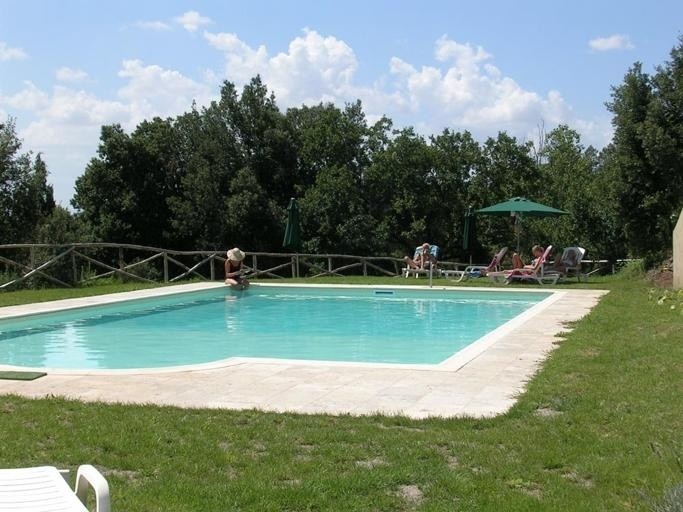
[512,244,545,275]
[225,247,249,287]
[404,242,436,271]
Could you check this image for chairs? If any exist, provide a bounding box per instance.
[401,245,587,287]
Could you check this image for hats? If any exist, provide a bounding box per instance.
[226,249,246,263]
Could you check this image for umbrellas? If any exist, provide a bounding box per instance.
[463,206,475,266]
[474,196,571,252]
